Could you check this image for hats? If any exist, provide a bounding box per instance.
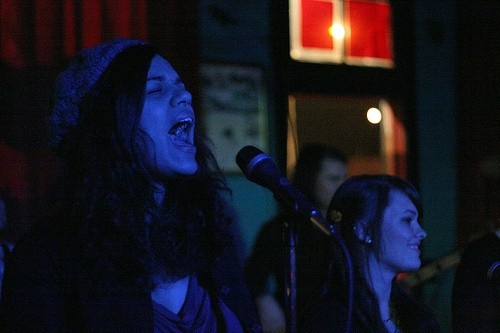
[36,39,145,155]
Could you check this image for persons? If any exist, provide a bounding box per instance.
[305,173,440,333]
[0,35,286,332]
[245,141,347,333]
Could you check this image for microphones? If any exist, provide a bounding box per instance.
[236,145,346,245]
[465,238,499,286]
